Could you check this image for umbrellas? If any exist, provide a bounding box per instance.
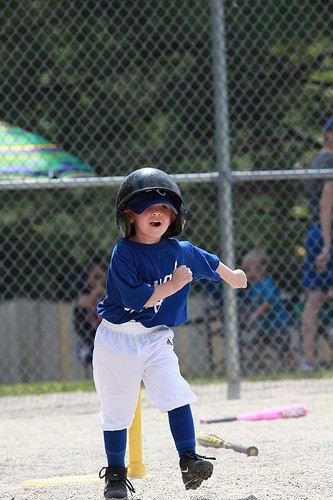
[2,122,96,183]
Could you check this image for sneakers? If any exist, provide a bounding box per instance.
[98,466,136,500]
[179,450,216,490]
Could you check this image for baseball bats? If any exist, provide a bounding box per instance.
[200,404,309,424]
[197,429,258,458]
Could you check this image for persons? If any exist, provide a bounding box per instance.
[299,116,332,372]
[237,251,291,371]
[91,167,248,499]
[74,267,107,380]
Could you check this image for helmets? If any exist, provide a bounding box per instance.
[115,167,186,239]
